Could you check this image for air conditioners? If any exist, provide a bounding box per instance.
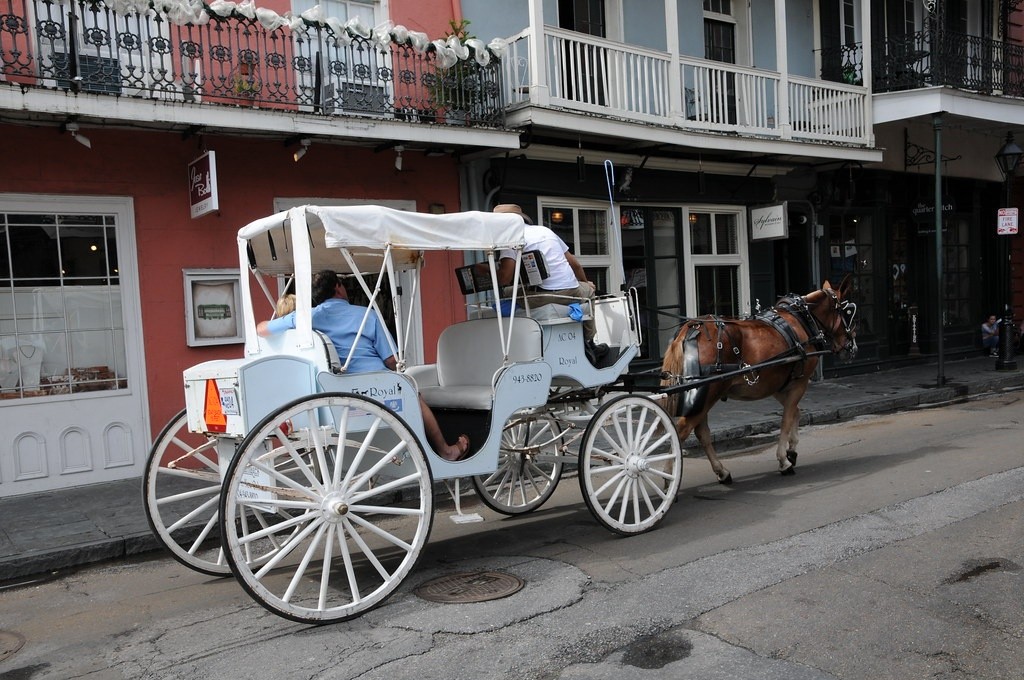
[324,75,395,120]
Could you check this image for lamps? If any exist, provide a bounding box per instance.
[425,147,447,158]
[688,214,697,224]
[394,145,405,171]
[552,212,564,223]
[293,138,312,162]
[65,123,92,148]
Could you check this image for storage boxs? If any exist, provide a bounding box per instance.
[183,358,248,439]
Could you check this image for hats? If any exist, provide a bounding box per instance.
[494,205,533,225]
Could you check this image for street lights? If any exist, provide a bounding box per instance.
[994,131,1024,372]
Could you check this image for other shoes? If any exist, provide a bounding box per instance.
[989,352,1000,357]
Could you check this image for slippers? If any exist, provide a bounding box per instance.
[455,434,470,460]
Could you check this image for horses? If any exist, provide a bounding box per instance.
[652,273,858,503]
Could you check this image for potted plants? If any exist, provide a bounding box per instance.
[428,16,498,127]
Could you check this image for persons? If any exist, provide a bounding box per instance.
[982,314,1000,357]
[259,269,470,461]
[479,204,610,364]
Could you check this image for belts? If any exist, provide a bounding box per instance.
[529,286,560,292]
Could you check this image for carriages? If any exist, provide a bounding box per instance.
[142,205,857,625]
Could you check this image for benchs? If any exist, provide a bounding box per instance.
[465,293,594,326]
[237,327,418,435]
[404,315,544,411]
